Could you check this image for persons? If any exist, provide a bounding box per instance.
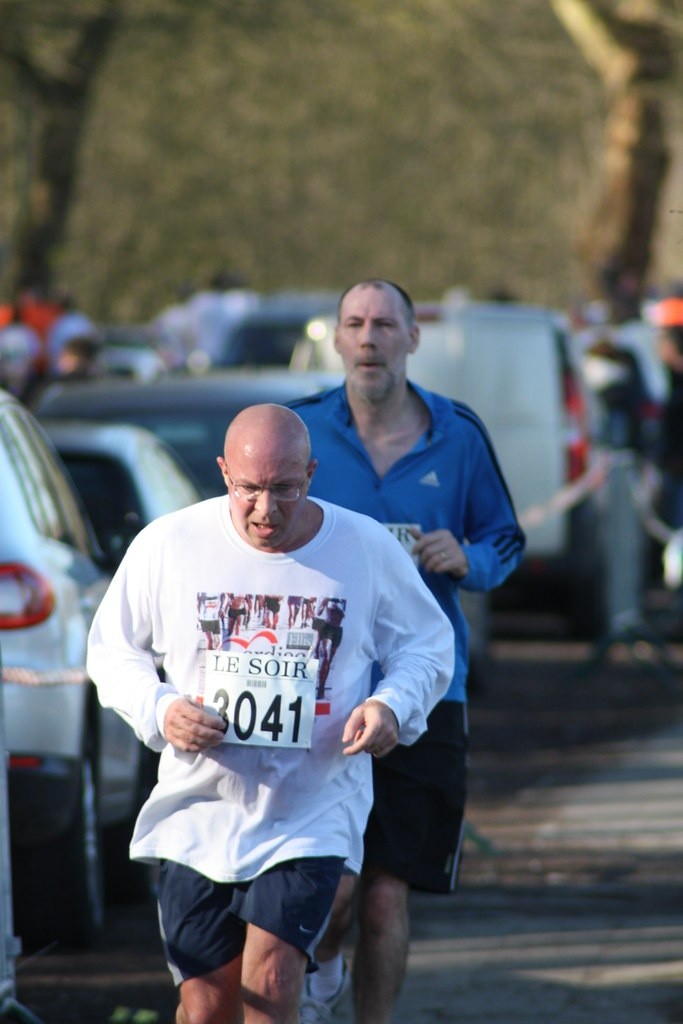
[87,404,457,1024]
[569,270,683,633]
[261,279,528,1024]
[0,266,265,429]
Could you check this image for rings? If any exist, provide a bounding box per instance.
[441,552,449,564]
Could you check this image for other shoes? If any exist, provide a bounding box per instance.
[299,953,347,1024]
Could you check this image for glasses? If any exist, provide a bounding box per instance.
[225,466,310,501]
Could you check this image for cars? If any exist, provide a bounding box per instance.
[0,292,671,950]
